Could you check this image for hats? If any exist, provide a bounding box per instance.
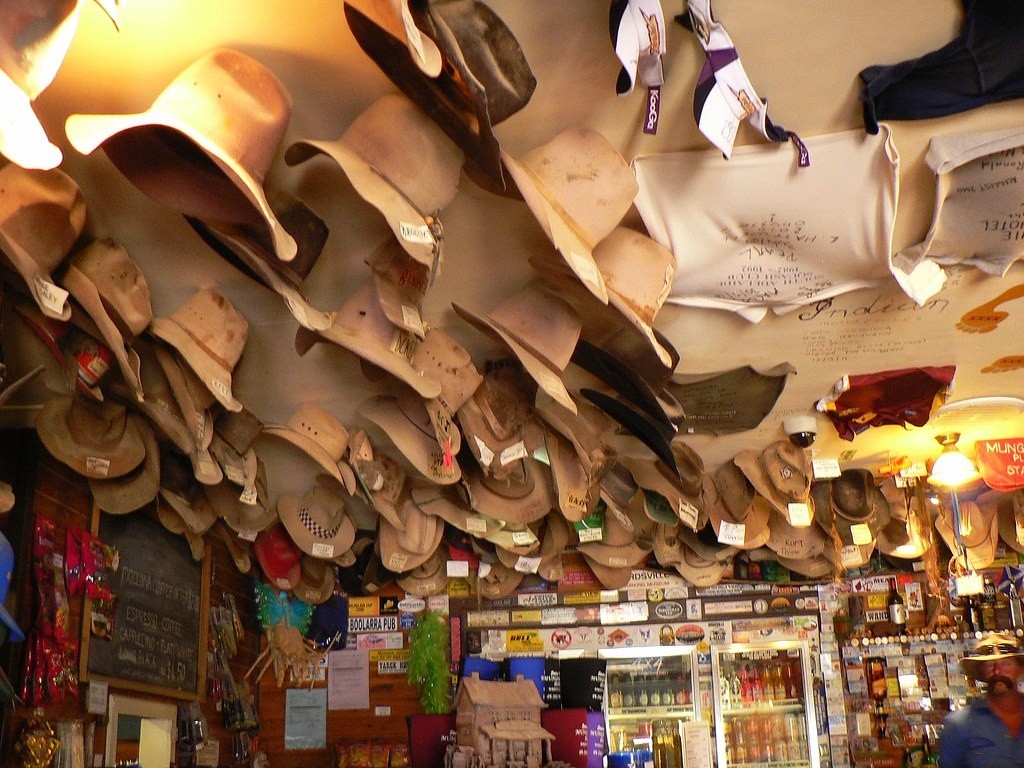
[0,0,1024,599]
[959,634,1024,681]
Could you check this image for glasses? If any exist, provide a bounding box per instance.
[974,644,1023,655]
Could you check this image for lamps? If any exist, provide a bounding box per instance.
[926,432,982,487]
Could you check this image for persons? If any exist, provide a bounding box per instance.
[941,634,1024,768]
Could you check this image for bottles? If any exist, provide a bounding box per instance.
[612,676,687,706]
[721,666,799,698]
[889,580,906,633]
[965,598,980,633]
